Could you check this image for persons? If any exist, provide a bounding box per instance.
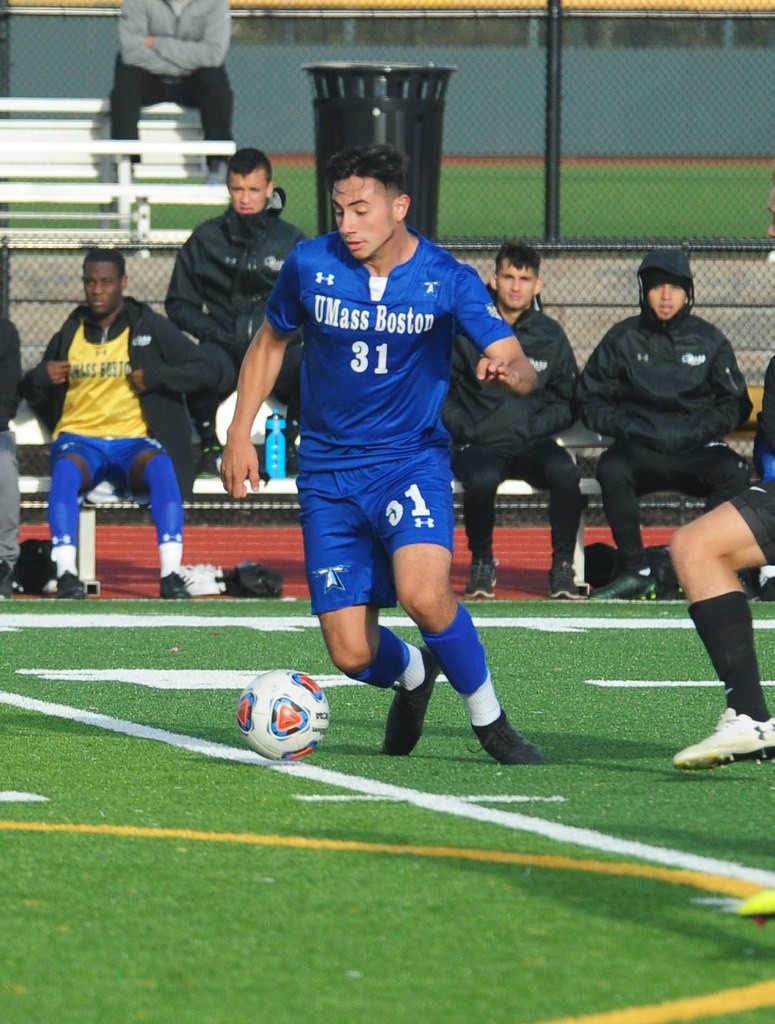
[165,148,305,474]
[220,144,544,765]
[441,244,581,599]
[20,249,220,599]
[0,318,22,601]
[752,359,775,602]
[108,0,234,184]
[575,248,754,597]
[667,186,775,770]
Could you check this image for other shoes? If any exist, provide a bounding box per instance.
[758,566,775,600]
[285,444,299,478]
[196,446,222,478]
[0,561,13,599]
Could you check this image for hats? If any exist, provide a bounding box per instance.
[642,267,690,303]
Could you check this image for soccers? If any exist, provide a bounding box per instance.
[237,668,330,762]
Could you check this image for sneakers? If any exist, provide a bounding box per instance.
[383,646,441,755]
[549,568,581,600]
[464,560,497,597]
[588,570,657,600]
[57,570,86,599]
[673,708,775,769]
[468,709,545,766]
[159,572,194,598]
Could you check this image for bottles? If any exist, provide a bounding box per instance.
[261,408,288,480]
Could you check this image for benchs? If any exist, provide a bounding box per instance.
[9,382,708,601]
[0,96,237,235]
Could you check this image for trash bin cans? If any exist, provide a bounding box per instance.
[299,62,458,239]
